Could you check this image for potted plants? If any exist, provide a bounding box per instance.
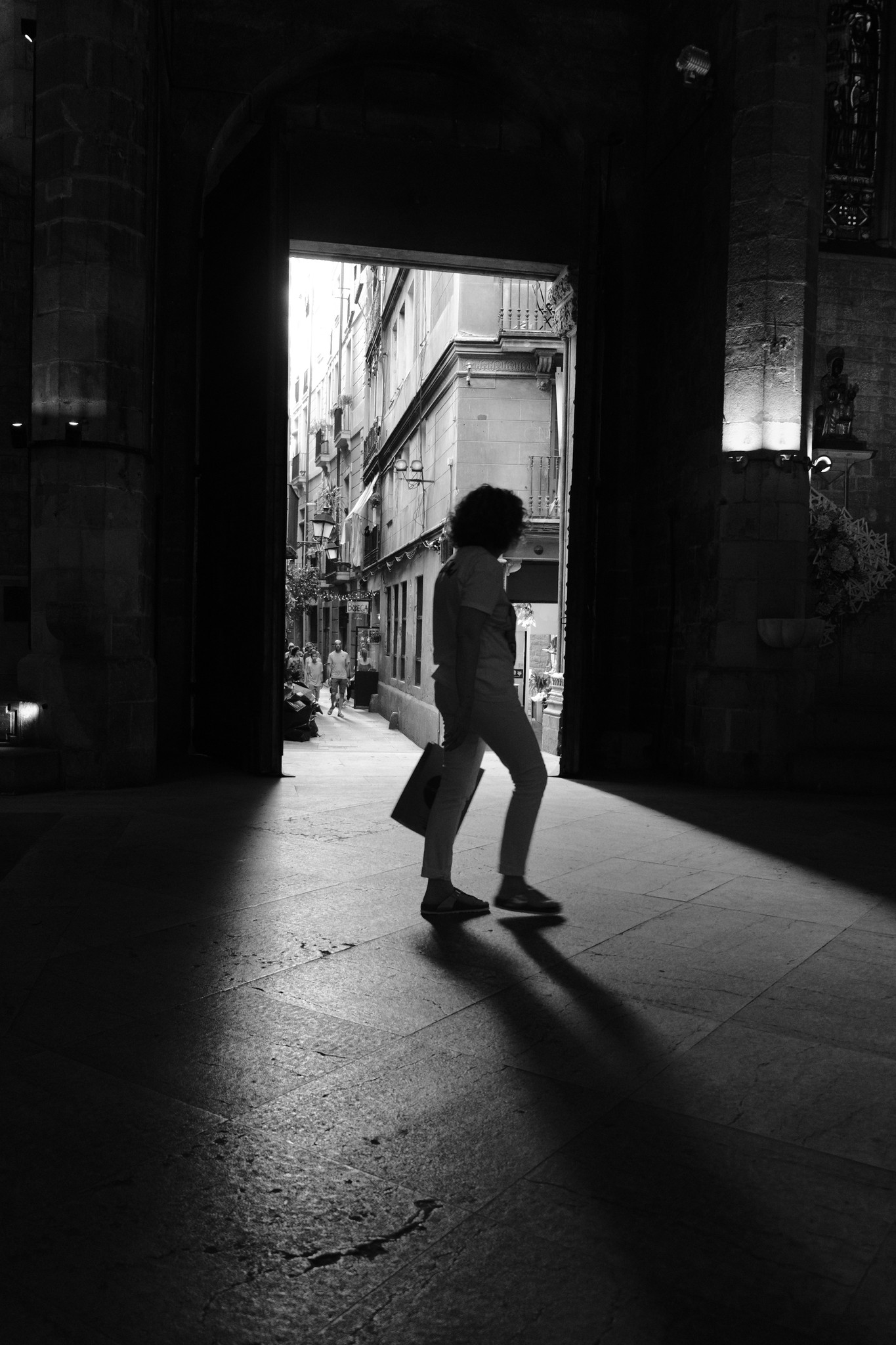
[529,670,549,728]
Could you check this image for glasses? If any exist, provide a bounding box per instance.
[307,646,311,649]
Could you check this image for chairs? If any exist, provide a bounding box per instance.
[292,703,322,739]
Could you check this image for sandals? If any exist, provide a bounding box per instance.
[338,713,344,717]
[327,705,335,715]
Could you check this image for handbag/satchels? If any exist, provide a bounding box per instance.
[391,741,485,839]
[292,671,300,679]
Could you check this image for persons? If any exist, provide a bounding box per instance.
[821,347,857,439]
[285,639,371,717]
[420,484,561,917]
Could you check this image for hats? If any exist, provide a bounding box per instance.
[288,642,294,650]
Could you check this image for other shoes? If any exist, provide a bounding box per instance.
[343,701,350,706]
[335,703,338,707]
[420,884,489,915]
[495,877,562,914]
